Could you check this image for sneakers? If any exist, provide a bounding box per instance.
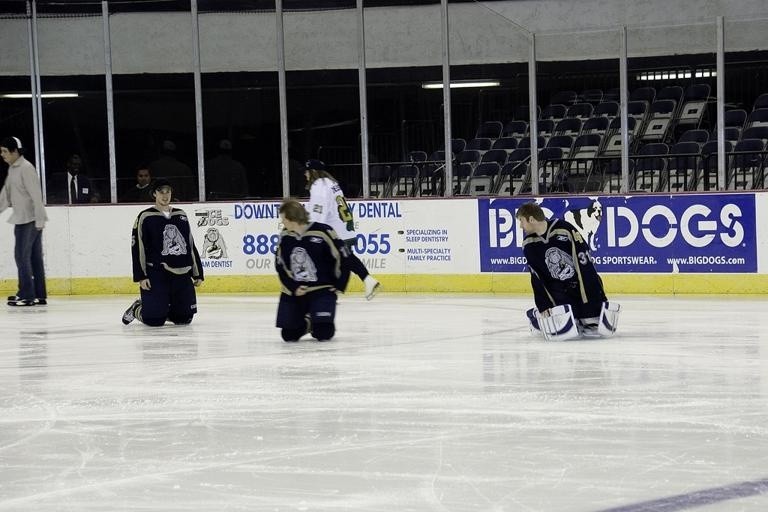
[364,274,380,297]
[7,295,47,307]
[122,299,141,324]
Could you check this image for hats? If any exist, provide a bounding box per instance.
[0,136,22,148]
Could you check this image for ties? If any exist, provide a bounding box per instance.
[69,176,79,205]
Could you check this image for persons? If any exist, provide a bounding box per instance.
[302,155,381,301]
[275,199,351,342]
[206,139,251,202]
[148,140,198,202]
[47,150,102,203]
[122,164,180,202]
[0,134,47,306]
[121,176,205,327]
[515,202,621,343]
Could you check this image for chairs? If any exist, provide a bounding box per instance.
[725,93,768,191]
[451,106,531,195]
[537,89,622,194]
[627,86,718,193]
[369,150,445,198]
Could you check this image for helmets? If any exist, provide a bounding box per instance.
[149,177,175,203]
[304,160,327,170]
